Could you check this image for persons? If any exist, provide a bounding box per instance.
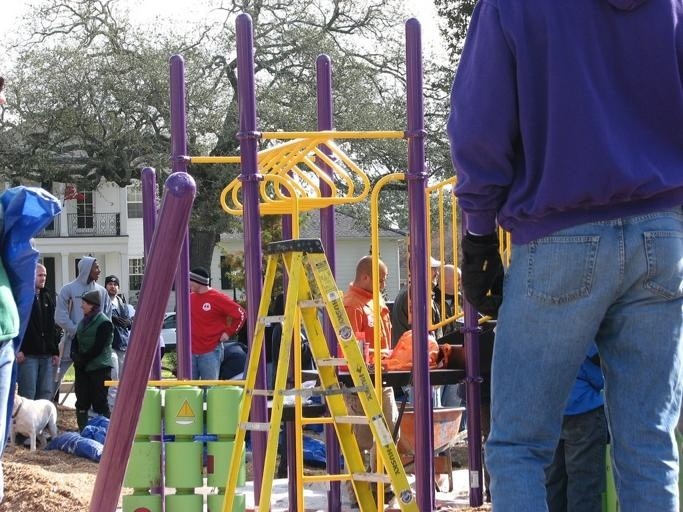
[53,257,112,393]
[392,257,497,443]
[543,337,609,512]
[446,1,683,512]
[69,289,114,431]
[189,266,245,380]
[105,274,132,380]
[272,292,311,391]
[341,257,394,388]
[18,262,60,402]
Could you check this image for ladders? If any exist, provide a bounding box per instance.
[222,239,420,512]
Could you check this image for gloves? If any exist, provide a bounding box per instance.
[461,230,503,318]
[111,314,131,329]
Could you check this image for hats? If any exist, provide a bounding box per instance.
[431,256,440,269]
[104,275,119,289]
[82,289,100,304]
[189,266,210,286]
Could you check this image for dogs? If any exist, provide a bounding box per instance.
[8,381,57,454]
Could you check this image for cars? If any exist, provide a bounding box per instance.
[161,311,179,347]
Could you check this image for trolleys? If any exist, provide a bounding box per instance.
[382,402,466,493]
